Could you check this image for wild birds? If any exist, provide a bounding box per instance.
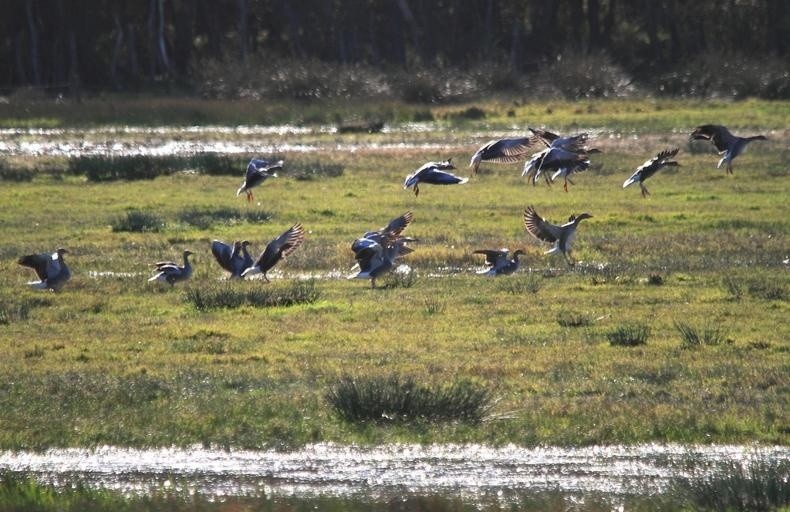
[402,158,470,198]
[622,146,684,199]
[15,248,70,293]
[687,124,770,175]
[210,222,305,285]
[146,249,197,289]
[470,126,604,193]
[471,246,527,278]
[522,202,595,267]
[346,210,419,288]
[235,156,284,205]
